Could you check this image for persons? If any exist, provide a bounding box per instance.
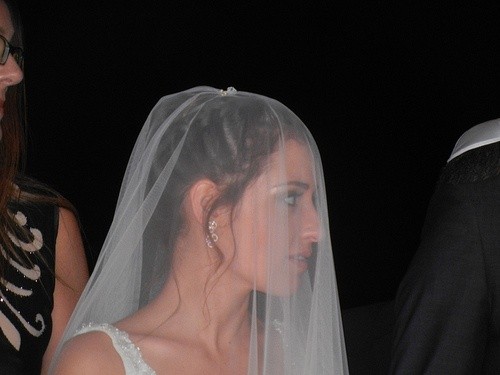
[0,1,90,375]
[46,86,349,375]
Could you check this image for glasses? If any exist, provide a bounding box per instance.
[0,34,24,70]
